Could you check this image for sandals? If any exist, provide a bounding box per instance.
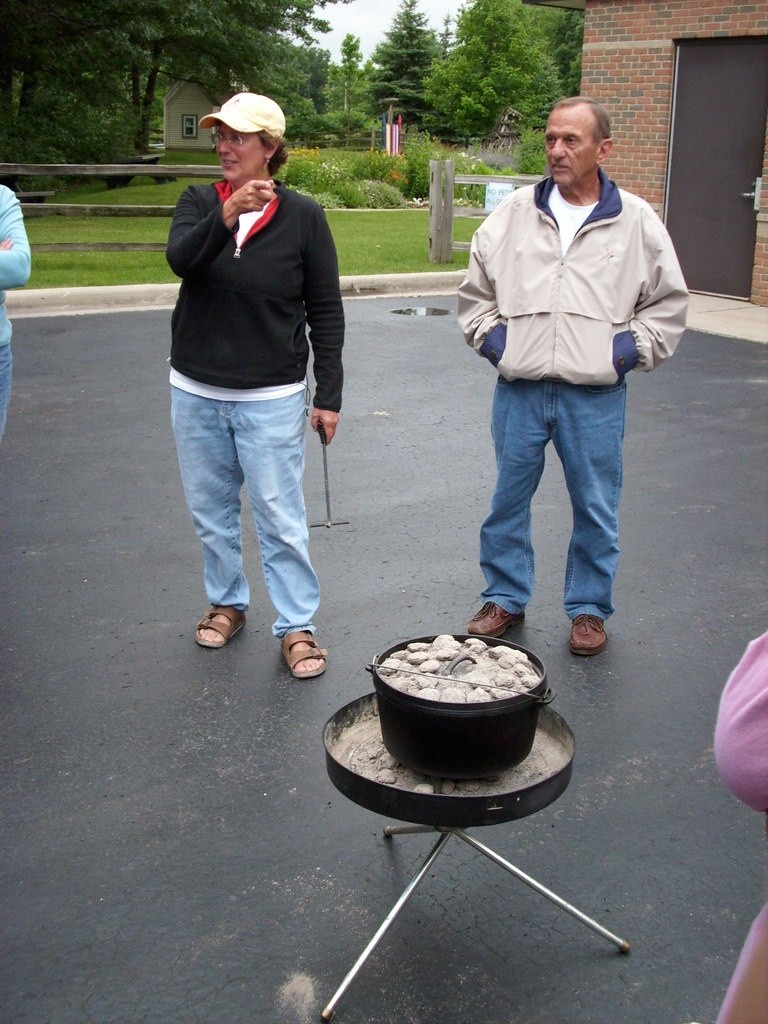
[280,633,327,680]
[195,602,246,648]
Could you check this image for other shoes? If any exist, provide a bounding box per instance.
[569,613,608,656]
[467,602,525,637]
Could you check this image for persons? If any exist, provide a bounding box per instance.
[458,97,689,654]
[166,92,345,677]
[715,631,768,1024]
[0,185,31,444]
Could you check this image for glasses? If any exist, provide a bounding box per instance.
[210,133,264,147]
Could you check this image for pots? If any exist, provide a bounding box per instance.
[365,633,557,780]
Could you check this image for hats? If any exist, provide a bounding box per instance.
[197,92,286,141]
